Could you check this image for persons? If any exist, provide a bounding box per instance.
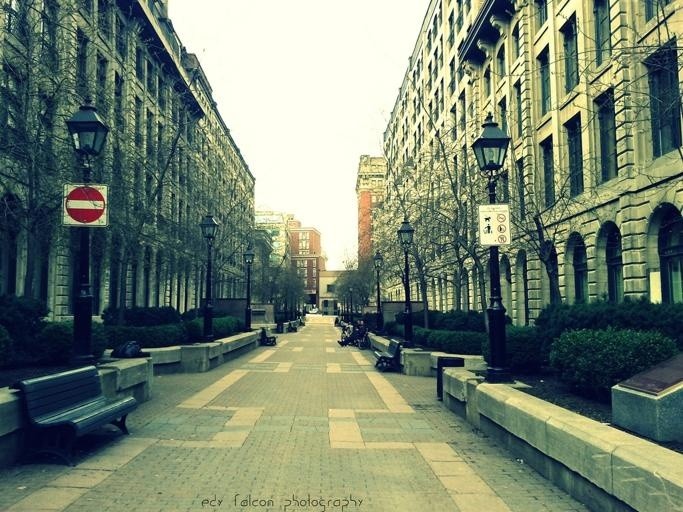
[337,320,367,347]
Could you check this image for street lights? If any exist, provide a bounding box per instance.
[199,209,219,343]
[242,241,256,331]
[372,249,385,335]
[66,94,112,369]
[395,214,415,347]
[471,112,514,385]
[342,284,355,325]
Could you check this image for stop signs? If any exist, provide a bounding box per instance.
[61,182,110,228]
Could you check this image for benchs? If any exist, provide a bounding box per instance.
[288,316,305,333]
[10,366,137,466]
[373,339,402,372]
[260,326,278,345]
